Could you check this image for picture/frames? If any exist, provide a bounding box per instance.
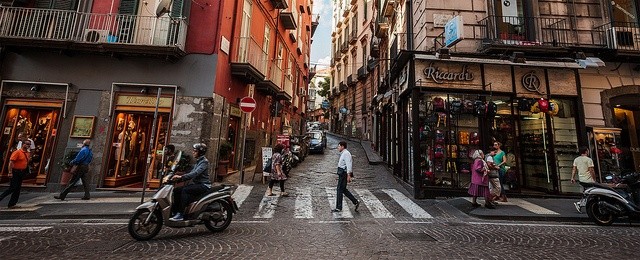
[69,115,95,138]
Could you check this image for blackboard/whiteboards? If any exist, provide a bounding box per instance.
[262,146,273,176]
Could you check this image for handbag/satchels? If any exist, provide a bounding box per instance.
[25,166,30,174]
[488,169,500,178]
[263,157,272,173]
[70,165,80,175]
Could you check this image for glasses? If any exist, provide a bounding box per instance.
[24,143,31,146]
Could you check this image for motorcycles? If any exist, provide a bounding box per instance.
[283,135,309,174]
[127,149,239,241]
[579,171,640,226]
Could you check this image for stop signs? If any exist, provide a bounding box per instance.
[239,96,256,113]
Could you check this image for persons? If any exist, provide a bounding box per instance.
[168,143,212,221]
[570,148,596,213]
[162,144,179,217]
[468,150,495,209]
[0,140,32,209]
[17,132,35,161]
[485,149,500,203]
[131,126,146,175]
[125,121,137,176]
[331,141,360,212]
[268,144,288,197]
[54,139,93,201]
[493,141,507,202]
[111,120,129,177]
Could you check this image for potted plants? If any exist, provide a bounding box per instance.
[218,142,232,175]
[61,149,78,185]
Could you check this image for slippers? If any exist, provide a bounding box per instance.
[268,193,276,196]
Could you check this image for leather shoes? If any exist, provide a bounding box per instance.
[54,194,65,200]
[331,208,341,212]
[355,200,361,212]
[82,197,90,200]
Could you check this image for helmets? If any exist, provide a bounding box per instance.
[193,143,207,156]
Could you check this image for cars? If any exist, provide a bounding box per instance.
[307,122,326,136]
[304,132,327,154]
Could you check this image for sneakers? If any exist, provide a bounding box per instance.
[471,203,481,207]
[486,201,494,206]
[573,201,582,214]
[169,213,184,220]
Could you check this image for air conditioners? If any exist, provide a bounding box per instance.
[82,29,109,43]
[298,88,307,96]
[605,27,639,51]
